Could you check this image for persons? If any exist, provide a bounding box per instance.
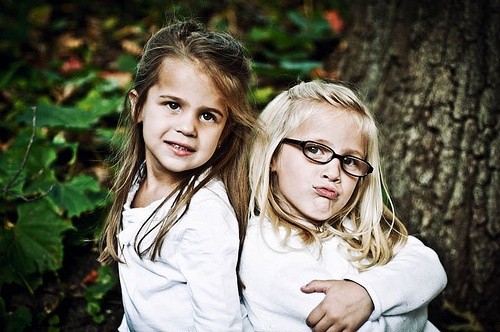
[236,80,449,332]
[105,19,253,332]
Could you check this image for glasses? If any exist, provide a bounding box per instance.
[281,138,373,178]
